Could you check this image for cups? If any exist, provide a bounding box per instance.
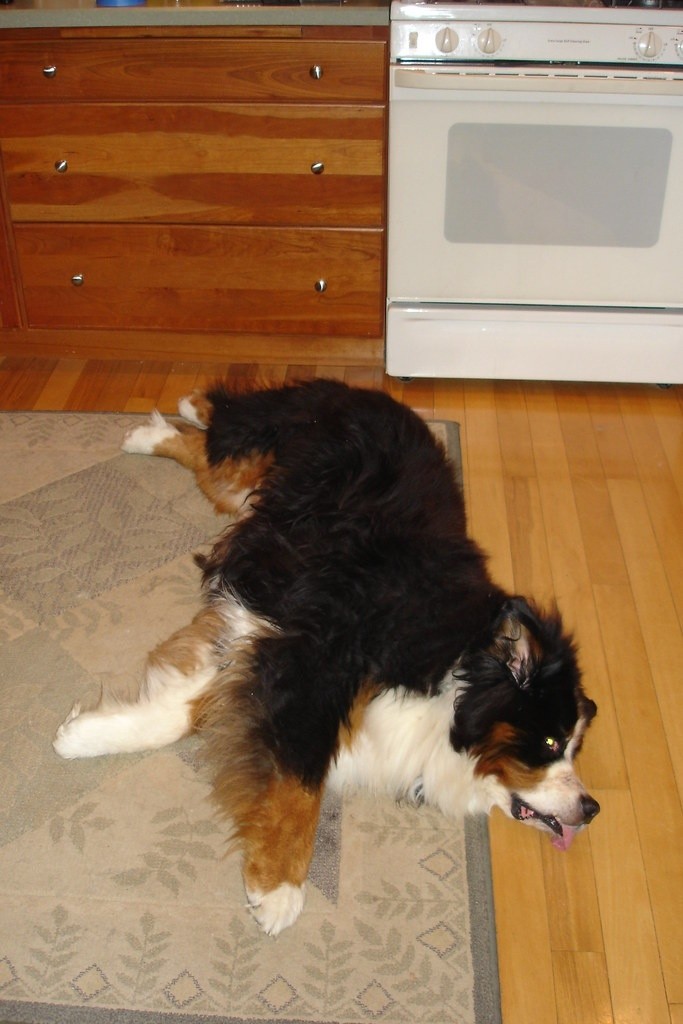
[97,0,146,7]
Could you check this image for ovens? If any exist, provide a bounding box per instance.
[386,2,683,385]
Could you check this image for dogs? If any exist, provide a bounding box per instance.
[51,375,605,941]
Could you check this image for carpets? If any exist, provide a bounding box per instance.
[0,412,504,1024]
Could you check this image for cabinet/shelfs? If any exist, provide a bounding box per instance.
[2,26,394,365]
